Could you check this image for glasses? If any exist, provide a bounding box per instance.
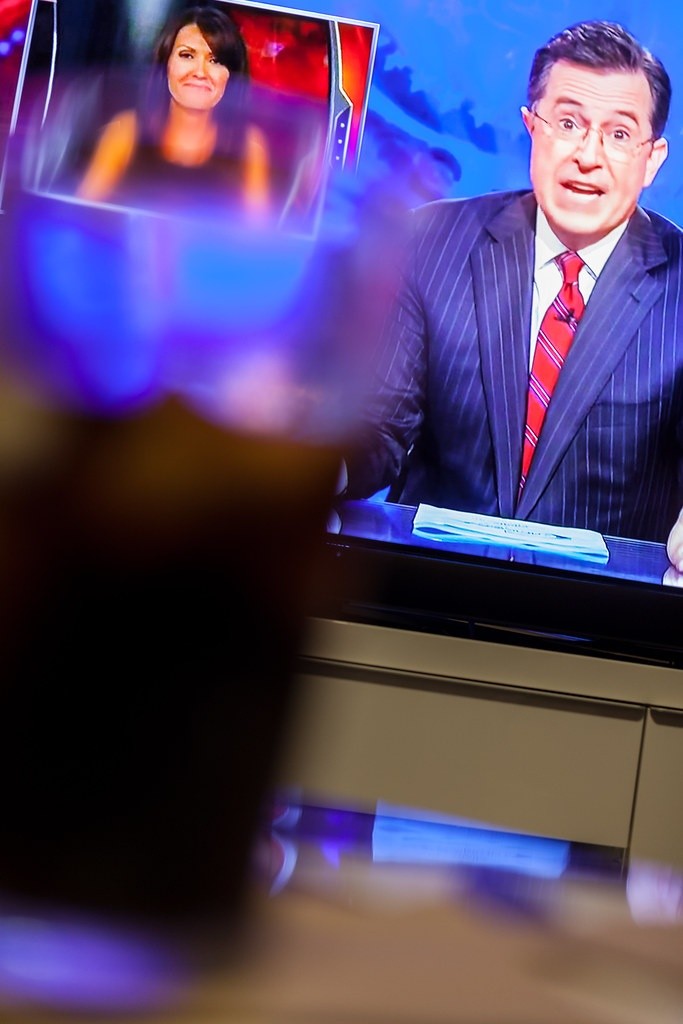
[532,103,655,164]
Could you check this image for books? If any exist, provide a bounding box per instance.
[413,502,609,558]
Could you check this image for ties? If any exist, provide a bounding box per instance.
[515,251,587,505]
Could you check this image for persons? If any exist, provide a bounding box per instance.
[330,20,683,576]
[75,6,267,229]
